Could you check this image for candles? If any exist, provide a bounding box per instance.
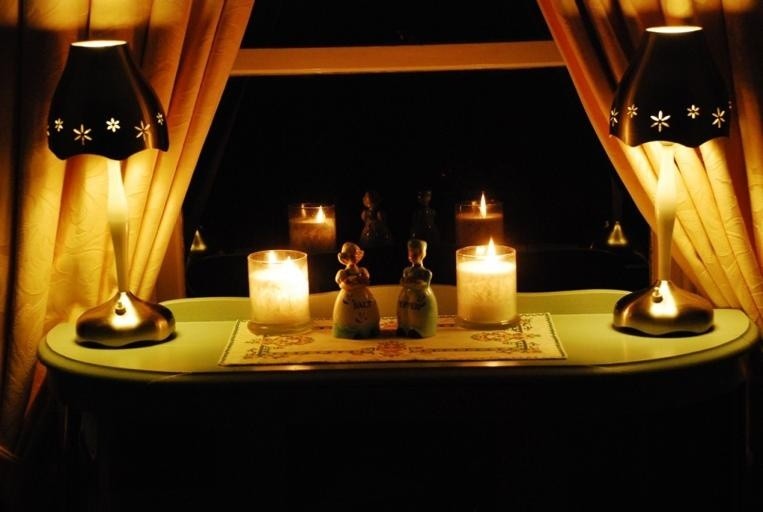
[288,201,335,255]
[456,245,518,331]
[248,250,310,334]
[456,193,505,249]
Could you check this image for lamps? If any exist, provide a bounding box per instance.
[607,25,732,336]
[44,40,176,348]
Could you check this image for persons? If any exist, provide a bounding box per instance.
[332,241,380,339]
[397,239,438,338]
[409,188,438,238]
[359,191,390,243]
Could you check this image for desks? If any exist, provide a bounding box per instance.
[13,288,763,511]
